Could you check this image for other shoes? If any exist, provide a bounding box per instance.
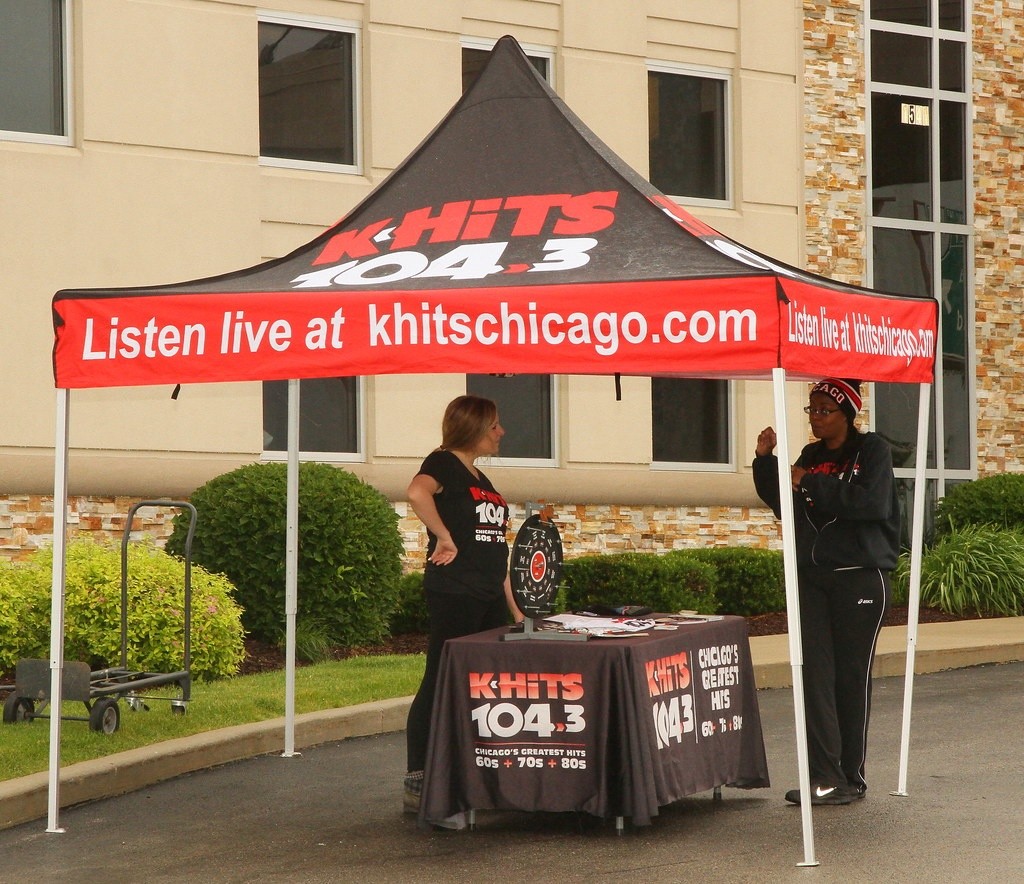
[403,773,424,813]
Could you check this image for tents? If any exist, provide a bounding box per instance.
[48,31,937,865]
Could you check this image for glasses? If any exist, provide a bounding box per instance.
[803,405,843,416]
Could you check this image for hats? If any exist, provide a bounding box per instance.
[809,378,863,423]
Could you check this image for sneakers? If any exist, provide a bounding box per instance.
[785,782,868,804]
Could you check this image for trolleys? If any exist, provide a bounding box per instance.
[2,501,197,732]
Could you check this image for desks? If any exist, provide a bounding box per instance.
[418,610,772,837]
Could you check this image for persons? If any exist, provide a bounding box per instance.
[403,395,528,812]
[753,376,903,805]
